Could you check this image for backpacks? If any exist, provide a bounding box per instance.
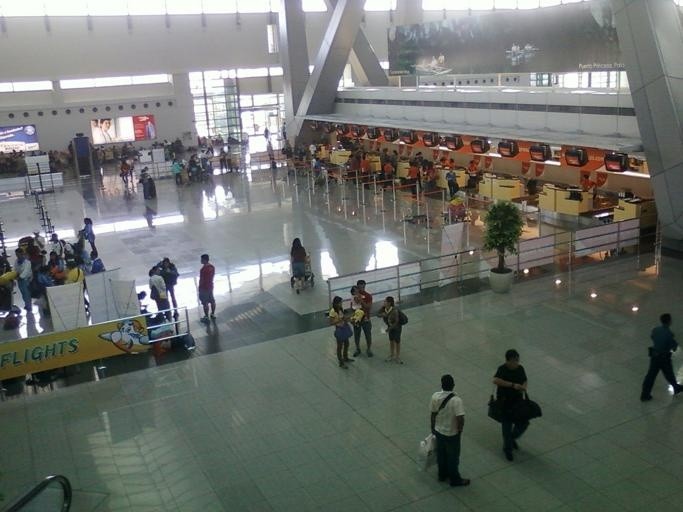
[150,284,160,300]
[398,311,408,324]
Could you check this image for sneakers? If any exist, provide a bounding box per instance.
[365,348,372,358]
[199,316,210,323]
[351,349,361,357]
[503,444,513,461]
[512,440,518,448]
[209,314,216,319]
[338,362,348,369]
[639,395,653,402]
[295,288,299,295]
[394,357,403,365]
[673,384,683,394]
[449,478,470,486]
[343,358,354,362]
[438,475,449,481]
[384,356,393,362]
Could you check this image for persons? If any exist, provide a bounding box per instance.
[641,314,682,401]
[0,218,105,312]
[267,139,481,199]
[198,254,216,323]
[91,119,113,144]
[96,136,248,200]
[492,349,528,462]
[429,373,471,486]
[5,149,70,176]
[329,280,374,370]
[68,140,75,161]
[137,257,180,339]
[290,237,310,295]
[282,122,286,139]
[376,296,405,365]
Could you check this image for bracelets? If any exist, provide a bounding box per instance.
[512,383,513,387]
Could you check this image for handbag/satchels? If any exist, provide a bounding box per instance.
[648,346,655,358]
[487,389,542,423]
[416,433,438,472]
[333,323,353,341]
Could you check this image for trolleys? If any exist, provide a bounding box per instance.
[441,191,469,224]
[291,254,315,289]
[188,165,202,183]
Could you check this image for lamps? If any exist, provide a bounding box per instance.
[265,1,279,54]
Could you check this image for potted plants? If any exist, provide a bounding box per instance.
[481,201,525,292]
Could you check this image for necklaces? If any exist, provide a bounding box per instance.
[385,306,392,314]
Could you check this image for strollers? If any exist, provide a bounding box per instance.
[64,231,84,263]
